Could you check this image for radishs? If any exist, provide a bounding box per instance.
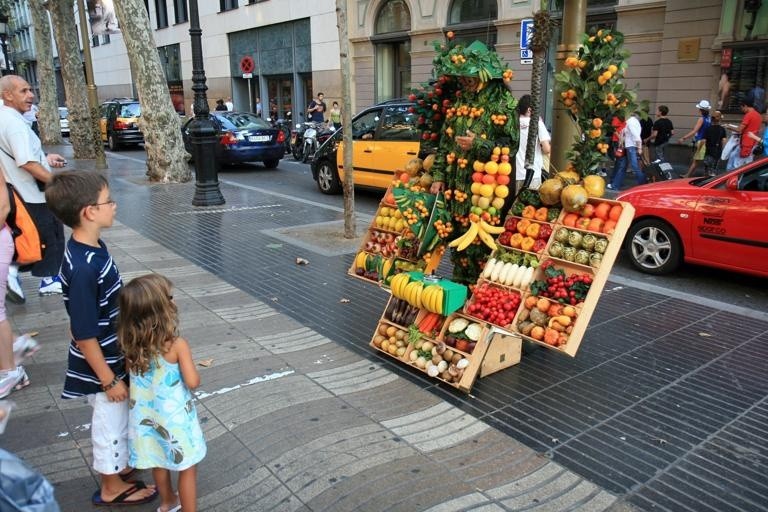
[482,259,534,289]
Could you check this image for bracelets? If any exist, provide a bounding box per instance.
[99,374,120,392]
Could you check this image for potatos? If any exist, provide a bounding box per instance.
[374,325,468,382]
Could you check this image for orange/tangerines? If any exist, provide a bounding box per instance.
[386,32,514,268]
[561,31,627,153]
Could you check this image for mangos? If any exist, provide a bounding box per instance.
[375,206,408,232]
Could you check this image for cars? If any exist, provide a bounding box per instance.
[58,107,69,133]
[179,111,286,170]
[611,156,768,276]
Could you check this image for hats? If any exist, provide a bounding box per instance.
[711,111,724,121]
[696,100,712,112]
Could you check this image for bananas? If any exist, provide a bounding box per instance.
[354,254,444,315]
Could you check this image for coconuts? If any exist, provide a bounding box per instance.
[538,169,605,214]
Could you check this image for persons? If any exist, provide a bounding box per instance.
[0,165,42,401]
[1,73,68,297]
[224,96,234,111]
[429,37,520,299]
[270,105,278,122]
[327,100,343,131]
[22,104,41,138]
[189,103,196,118]
[515,94,551,197]
[116,273,207,511]
[255,97,261,118]
[362,110,383,140]
[307,92,326,123]
[215,97,228,111]
[607,84,768,190]
[45,167,160,508]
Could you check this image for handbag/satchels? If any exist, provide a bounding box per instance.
[616,148,625,157]
[693,134,766,162]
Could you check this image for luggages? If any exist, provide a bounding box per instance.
[639,152,680,183]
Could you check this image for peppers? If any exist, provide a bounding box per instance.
[501,190,559,254]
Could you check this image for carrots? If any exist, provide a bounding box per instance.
[416,313,443,338]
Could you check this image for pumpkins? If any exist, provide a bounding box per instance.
[516,297,576,347]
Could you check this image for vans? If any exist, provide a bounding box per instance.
[98,97,144,151]
[311,98,421,195]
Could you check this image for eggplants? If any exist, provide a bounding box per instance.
[383,297,420,328]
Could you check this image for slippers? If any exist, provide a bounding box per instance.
[156,490,184,512]
[92,467,158,505]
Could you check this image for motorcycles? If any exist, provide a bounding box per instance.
[266,111,338,162]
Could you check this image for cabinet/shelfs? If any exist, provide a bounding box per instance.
[342,168,638,401]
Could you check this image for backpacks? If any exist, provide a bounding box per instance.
[5,182,43,265]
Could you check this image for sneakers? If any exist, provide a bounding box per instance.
[13,332,42,364]
[5,273,28,305]
[38,275,64,295]
[0,366,32,397]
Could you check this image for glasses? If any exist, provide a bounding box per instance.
[87,199,114,207]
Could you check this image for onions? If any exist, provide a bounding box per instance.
[364,232,400,255]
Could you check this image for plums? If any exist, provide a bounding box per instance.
[538,261,593,305]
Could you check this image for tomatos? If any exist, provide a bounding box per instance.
[467,287,521,327]
[563,203,623,234]
